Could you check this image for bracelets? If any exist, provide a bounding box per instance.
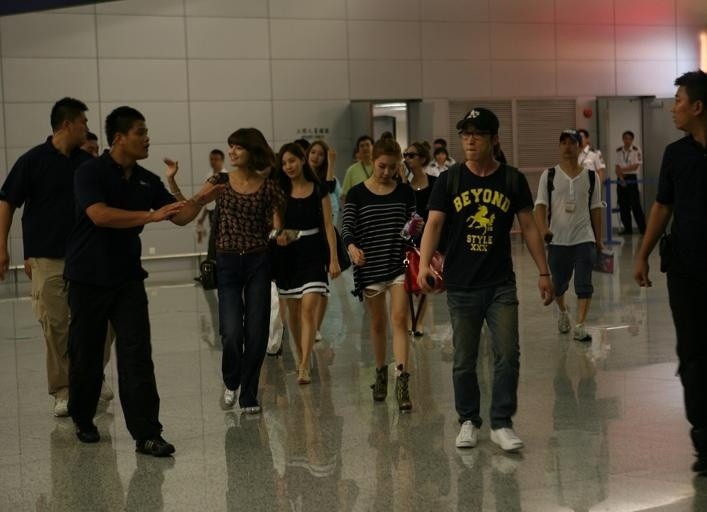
[171,189,182,197]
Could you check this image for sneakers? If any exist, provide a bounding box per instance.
[136,436,175,456]
[297,369,311,384]
[491,427,523,450]
[455,420,478,448]
[53,387,69,416]
[76,421,99,442]
[221,383,261,413]
[558,311,570,333]
[574,326,591,341]
[101,380,113,400]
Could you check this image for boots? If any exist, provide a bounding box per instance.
[370,365,388,400]
[396,372,412,410]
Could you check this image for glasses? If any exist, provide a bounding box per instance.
[458,131,490,139]
[404,152,420,159]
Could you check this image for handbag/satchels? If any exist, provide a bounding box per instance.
[194,259,217,290]
[404,246,445,293]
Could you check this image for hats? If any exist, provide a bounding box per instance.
[560,130,582,146]
[456,108,499,130]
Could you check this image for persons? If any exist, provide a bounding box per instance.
[0,96,116,417]
[635,67,707,477]
[342,136,430,413]
[162,128,285,414]
[534,126,648,345]
[67,105,230,456]
[298,136,375,341]
[196,149,283,353]
[271,142,341,385]
[404,138,457,335]
[414,105,555,452]
[80,127,102,158]
